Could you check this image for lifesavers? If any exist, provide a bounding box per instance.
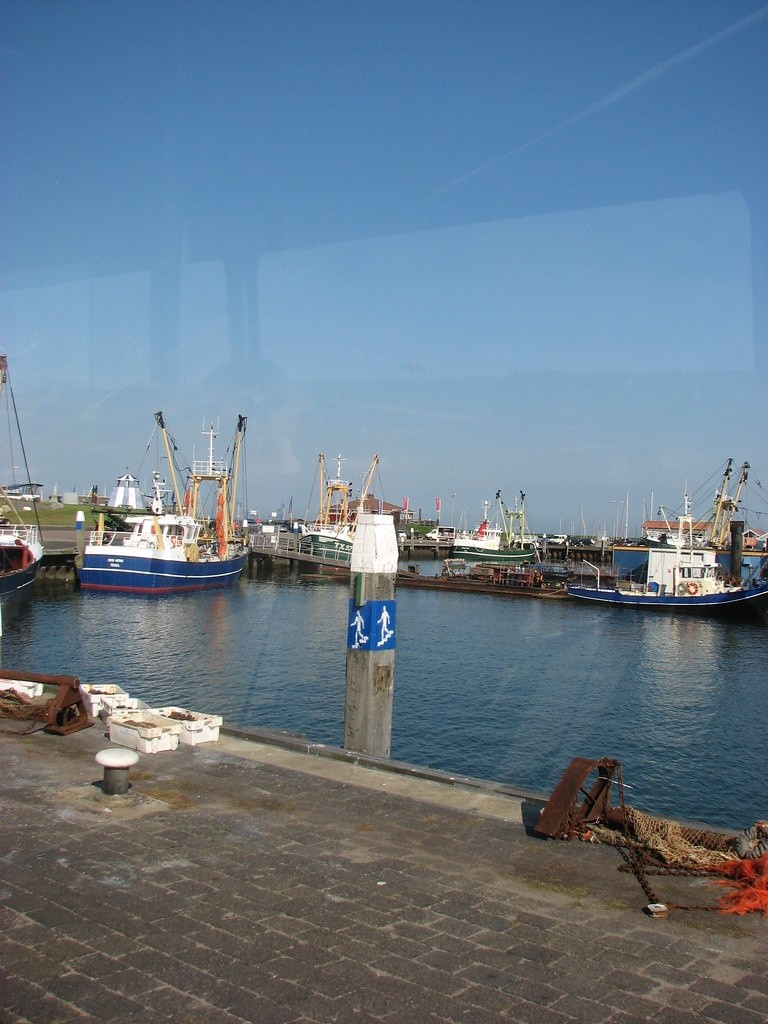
[171,536,176,544]
[687,583,698,595]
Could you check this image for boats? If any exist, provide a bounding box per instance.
[0,351,46,624]
[79,411,254,593]
[301,455,380,573]
[453,491,538,563]
[567,455,768,616]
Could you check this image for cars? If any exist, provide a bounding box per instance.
[250,524,765,549]
